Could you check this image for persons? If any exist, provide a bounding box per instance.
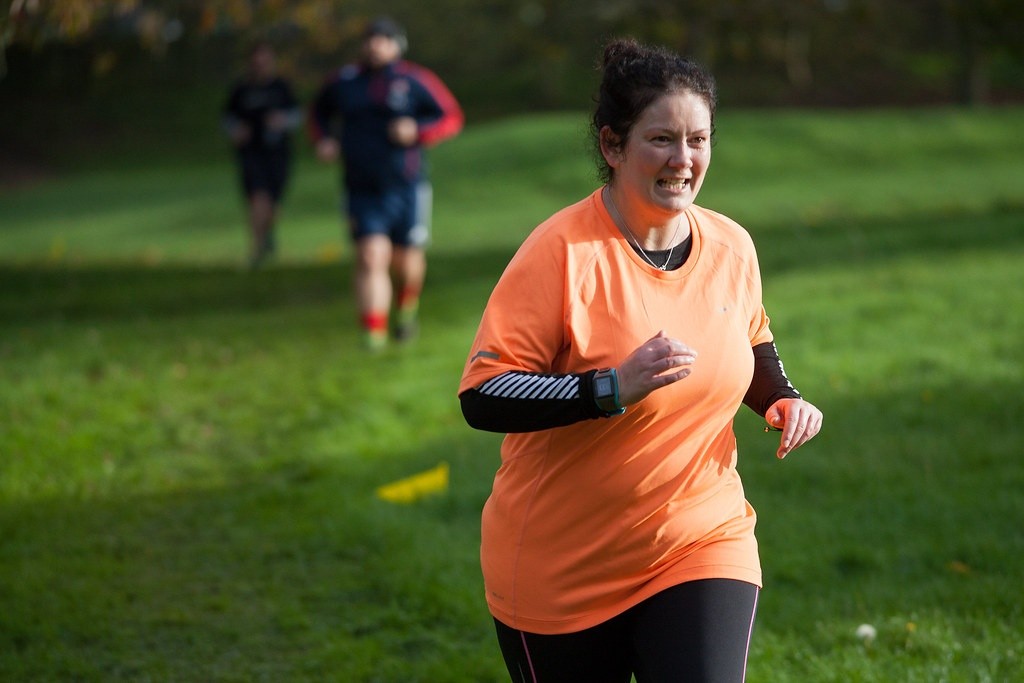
[458,40,823,682]
[217,41,309,259]
[310,21,462,342]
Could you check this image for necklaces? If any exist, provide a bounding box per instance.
[608,181,681,270]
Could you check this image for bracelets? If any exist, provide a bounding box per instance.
[764,426,783,434]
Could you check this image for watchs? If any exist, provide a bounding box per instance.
[594,368,627,416]
